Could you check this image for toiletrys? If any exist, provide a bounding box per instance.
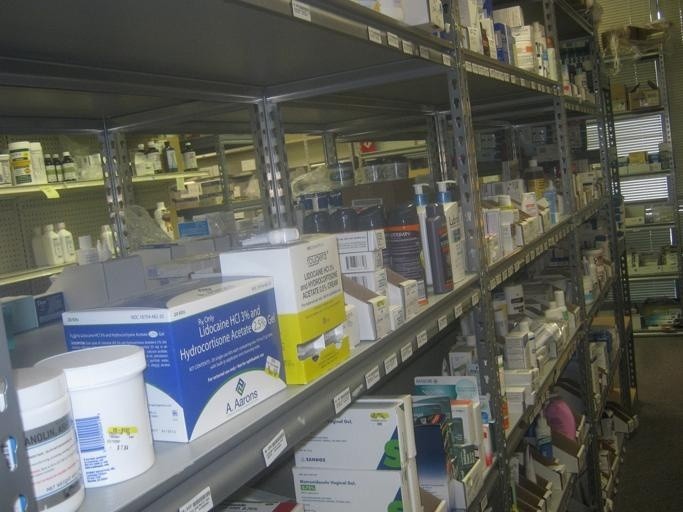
[291,179,468,290]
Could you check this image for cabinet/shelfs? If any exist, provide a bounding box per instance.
[0,0,682,511]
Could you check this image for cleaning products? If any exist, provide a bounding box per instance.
[33,219,75,266]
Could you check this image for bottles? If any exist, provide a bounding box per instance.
[32,222,76,266]
[519,321,538,369]
[183,142,198,172]
[101,225,115,255]
[545,290,570,341]
[535,409,553,462]
[546,36,559,83]
[574,62,587,90]
[162,141,179,173]
[62,151,76,181]
[525,159,546,201]
[76,235,100,266]
[137,141,163,174]
[44,153,64,183]
[154,201,175,242]
